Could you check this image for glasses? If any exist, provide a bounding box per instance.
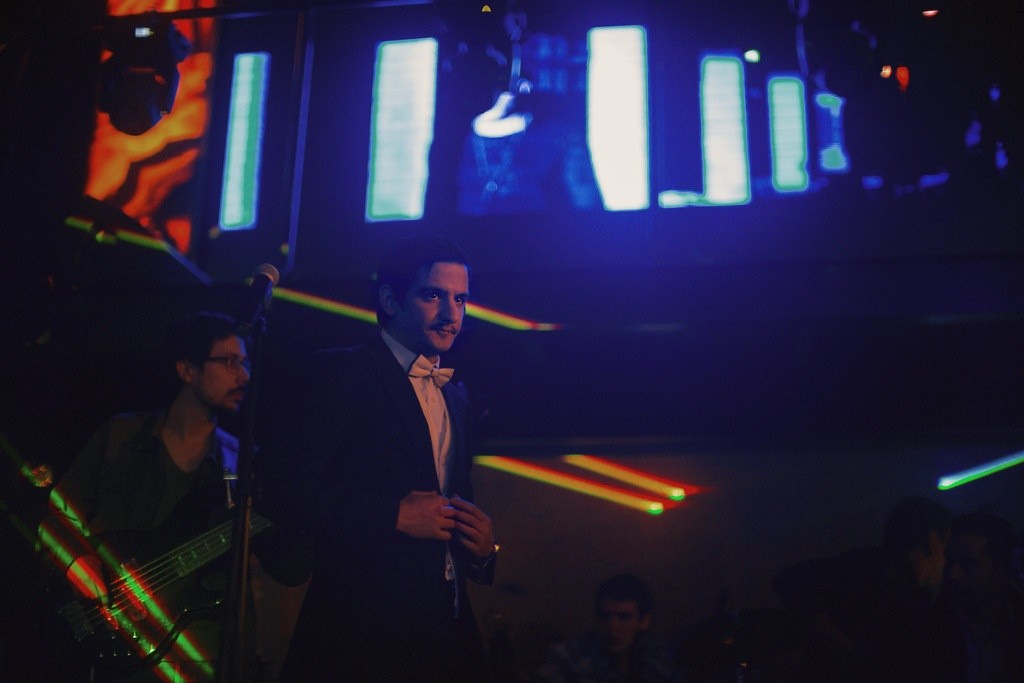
[188,353,252,374]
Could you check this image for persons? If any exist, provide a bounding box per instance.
[261,231,495,683]
[48,311,259,683]
[538,492,1023,682]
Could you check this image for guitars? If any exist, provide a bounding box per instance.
[58,483,273,678]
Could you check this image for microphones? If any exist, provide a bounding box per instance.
[235,263,280,339]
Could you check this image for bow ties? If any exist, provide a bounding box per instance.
[408,354,455,388]
